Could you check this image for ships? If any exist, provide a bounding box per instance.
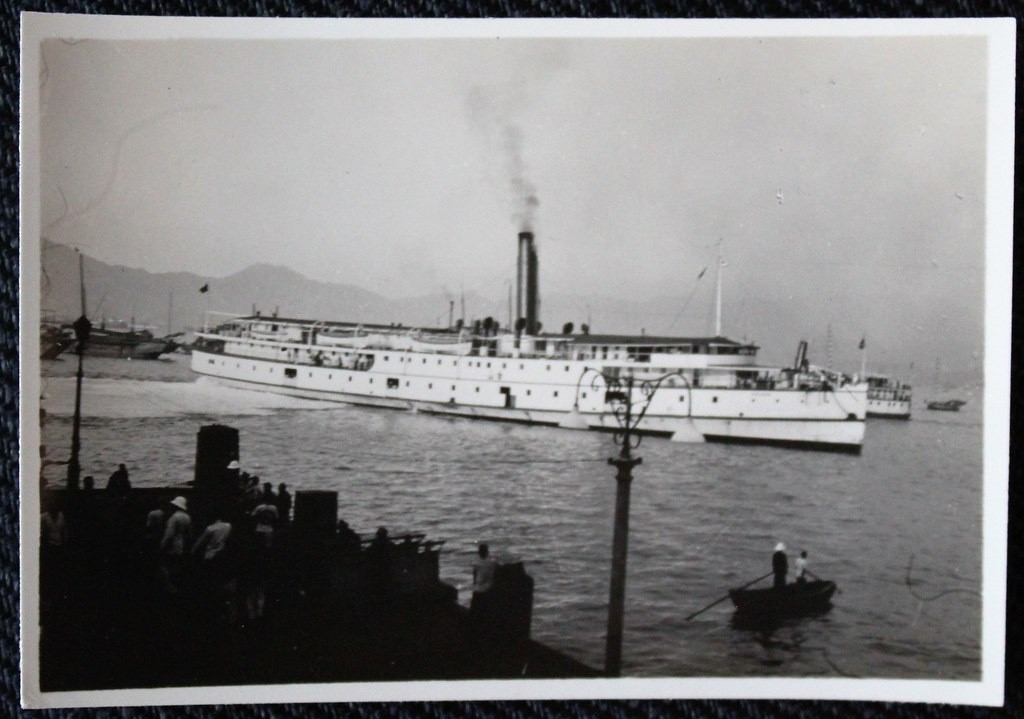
[190,232,869,450]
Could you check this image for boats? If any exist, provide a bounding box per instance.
[928,397,964,414]
[717,573,838,619]
[862,376,913,418]
[61,317,181,364]
[39,321,71,361]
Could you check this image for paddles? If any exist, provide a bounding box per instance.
[806,569,843,597]
[685,571,773,620]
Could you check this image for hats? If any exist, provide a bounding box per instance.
[227,459,240,471]
[774,541,786,553]
[171,496,189,510]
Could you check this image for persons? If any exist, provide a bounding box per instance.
[470,543,500,608]
[772,543,789,590]
[795,549,816,584]
[37,463,440,626]
